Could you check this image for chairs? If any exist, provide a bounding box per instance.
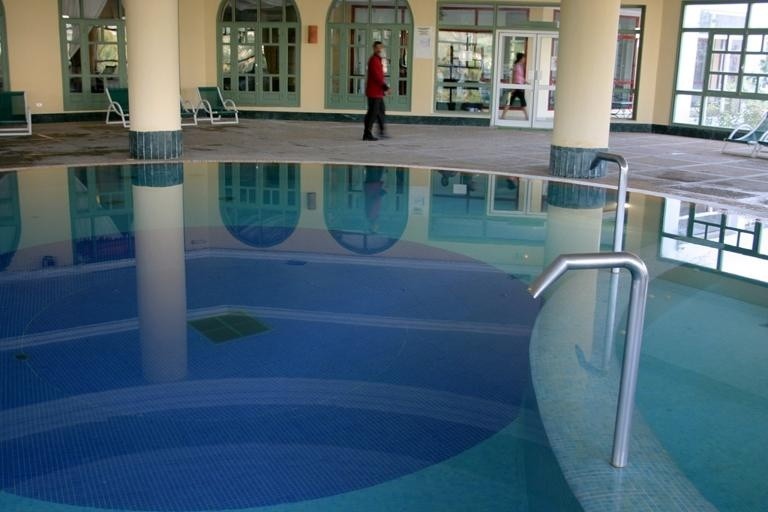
[180,95,197,126]
[194,85,239,125]
[105,87,130,127]
[721,110,768,156]
[756,129,768,158]
[1,90,32,136]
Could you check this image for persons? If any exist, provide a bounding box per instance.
[363,41,392,141]
[362,165,387,234]
[501,52,530,120]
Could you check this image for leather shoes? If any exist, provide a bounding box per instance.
[363,136,378,141]
